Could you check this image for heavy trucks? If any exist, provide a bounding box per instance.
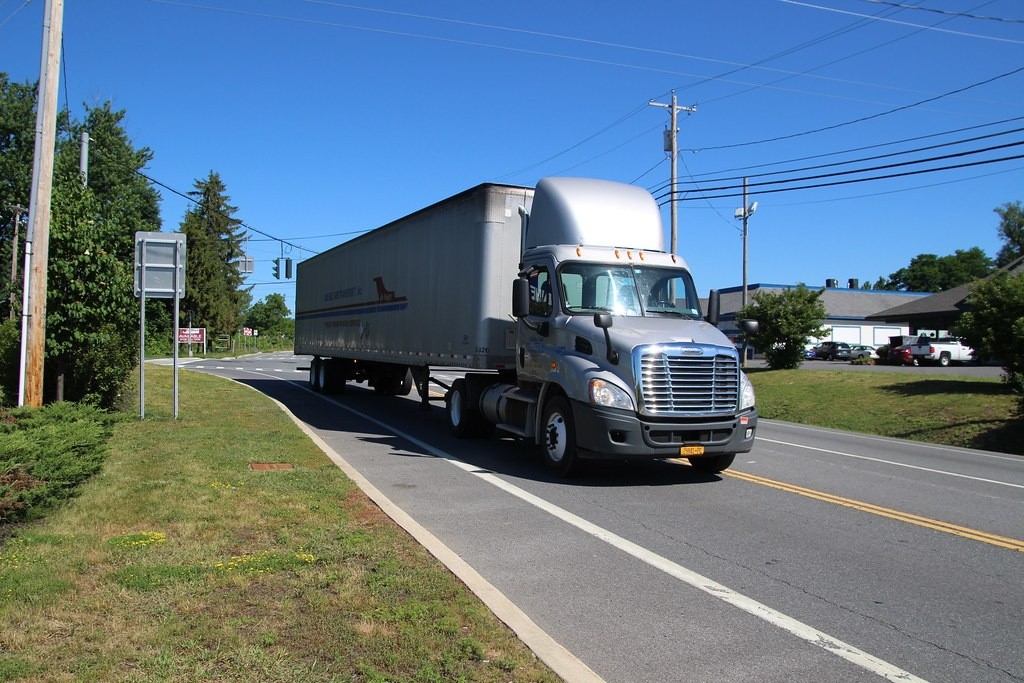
[293,176,758,474]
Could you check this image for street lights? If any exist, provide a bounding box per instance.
[9,204,29,318]
[734,176,758,314]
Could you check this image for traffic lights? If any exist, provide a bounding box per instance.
[272,258,280,279]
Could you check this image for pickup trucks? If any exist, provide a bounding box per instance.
[911,336,974,367]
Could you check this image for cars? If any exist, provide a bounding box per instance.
[876,344,920,366]
[849,345,880,359]
[803,349,816,361]
[810,341,851,361]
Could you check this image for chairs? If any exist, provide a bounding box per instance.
[616,285,647,307]
[540,279,570,311]
